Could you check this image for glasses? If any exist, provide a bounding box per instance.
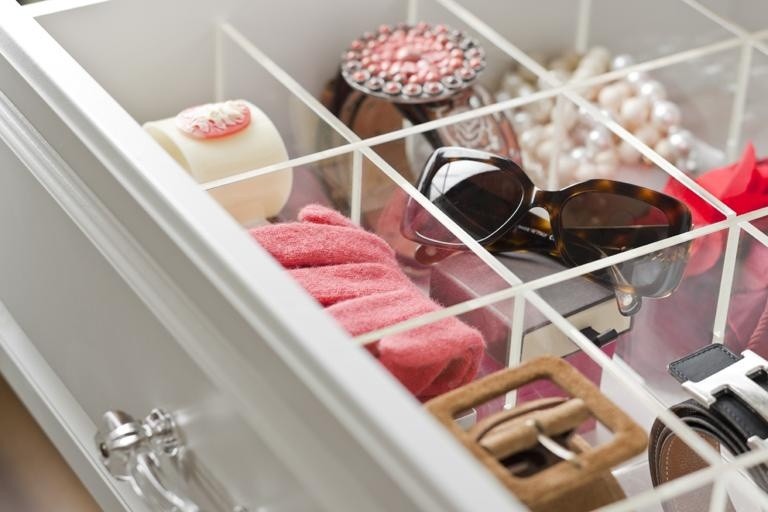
[399,146,693,317]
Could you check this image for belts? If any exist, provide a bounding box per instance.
[647,342,768,511]
[422,354,650,511]
[314,19,527,282]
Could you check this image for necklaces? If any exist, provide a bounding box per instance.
[492,44,698,184]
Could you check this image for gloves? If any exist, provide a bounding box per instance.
[247,202,487,404]
